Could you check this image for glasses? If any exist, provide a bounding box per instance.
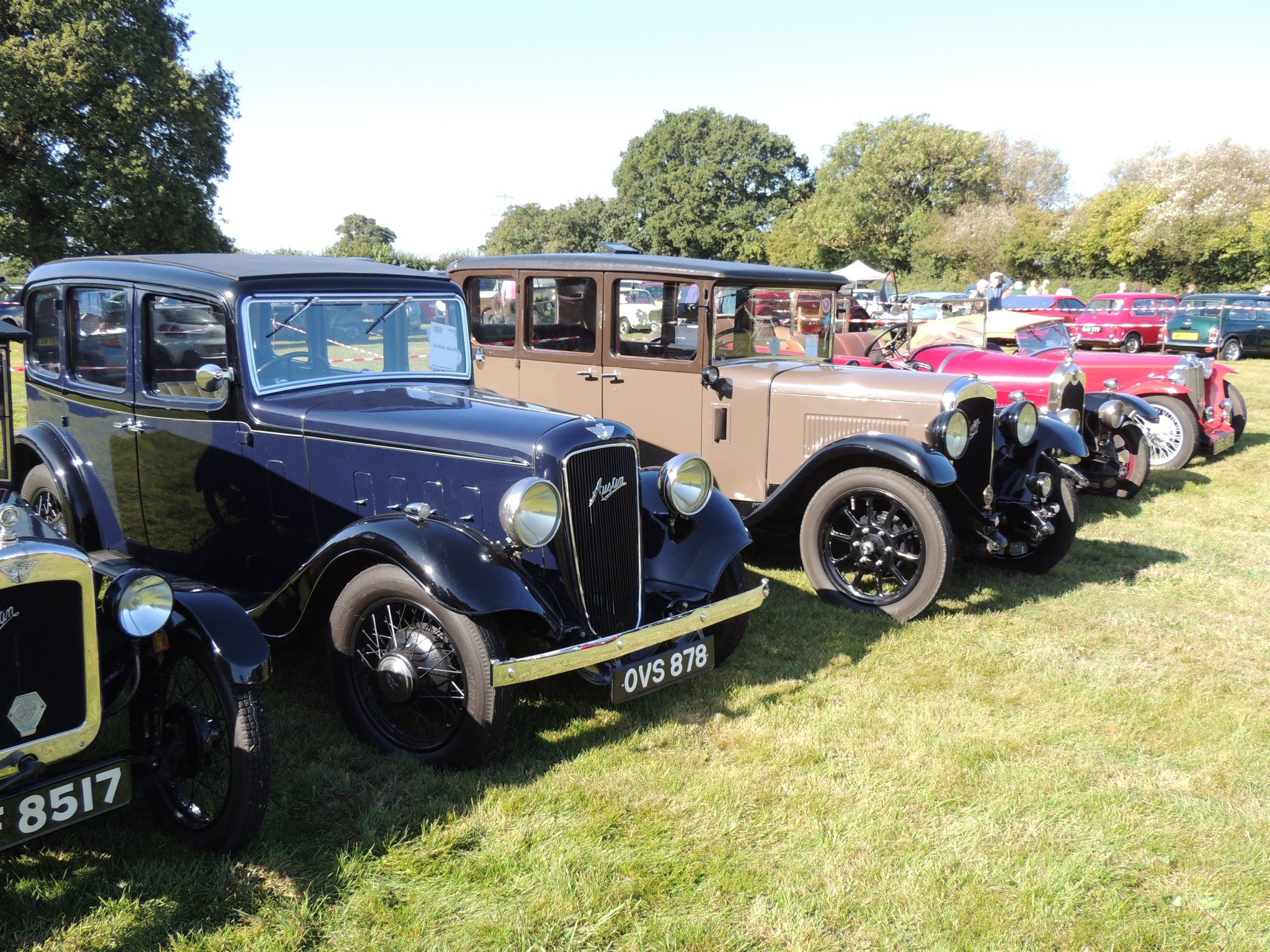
[995,277,1001,279]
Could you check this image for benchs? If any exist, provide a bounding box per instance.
[101,360,296,400]
[789,331,892,360]
[479,319,595,353]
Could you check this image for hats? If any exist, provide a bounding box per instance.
[1260,285,1270,292]
[1042,279,1050,284]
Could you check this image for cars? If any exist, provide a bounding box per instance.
[1,277,557,343]
[618,287,660,335]
[1072,292,1181,353]
[437,249,1094,619]
[10,250,771,766]
[628,268,1086,335]
[671,310,1246,499]
[1,320,270,888]
[1164,294,1270,361]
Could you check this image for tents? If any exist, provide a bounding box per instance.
[830,259,891,290]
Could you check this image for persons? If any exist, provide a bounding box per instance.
[1115,281,1157,306]
[685,284,699,303]
[500,280,516,324]
[715,287,724,314]
[1055,282,1072,297]
[1182,284,1195,307]
[1253,284,1270,308]
[965,271,1050,312]
